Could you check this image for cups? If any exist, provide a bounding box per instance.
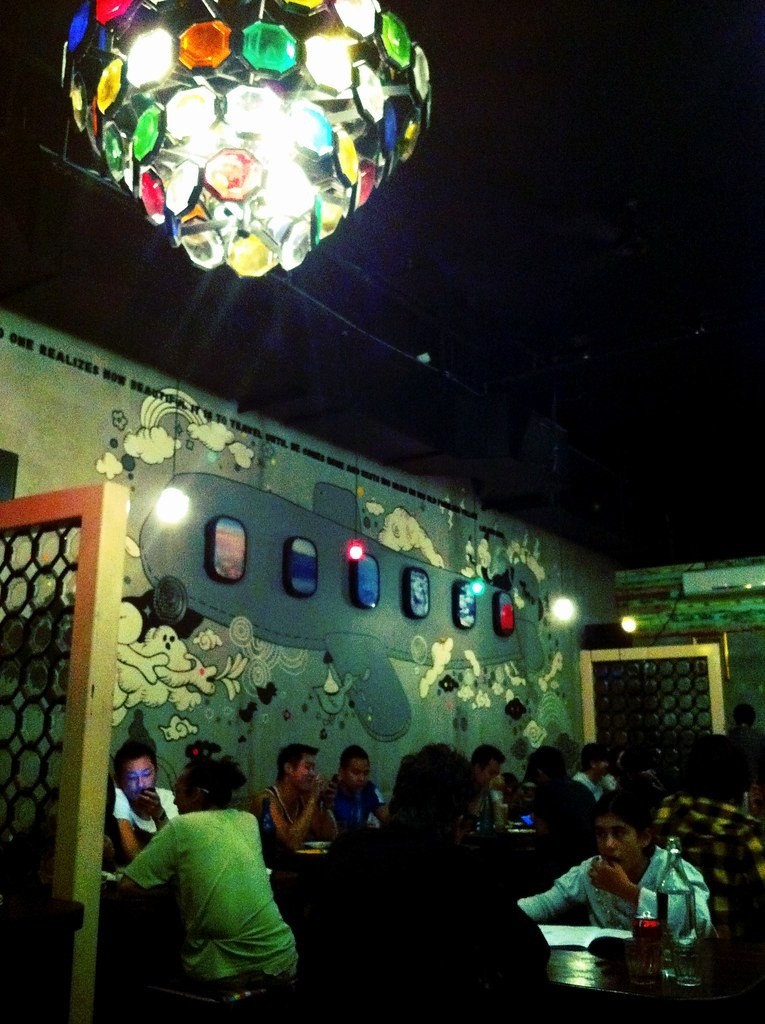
[626,940,659,985]
[670,938,704,986]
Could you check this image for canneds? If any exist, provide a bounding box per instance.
[633,912,658,977]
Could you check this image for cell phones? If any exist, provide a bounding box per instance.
[522,815,535,828]
[322,773,340,802]
[140,786,156,798]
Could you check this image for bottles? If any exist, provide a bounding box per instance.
[475,808,493,837]
[656,836,696,976]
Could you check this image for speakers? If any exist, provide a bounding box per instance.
[584,623,633,649]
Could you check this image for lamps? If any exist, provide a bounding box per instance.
[58,0,432,280]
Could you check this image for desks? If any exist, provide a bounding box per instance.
[537,924,765,1024]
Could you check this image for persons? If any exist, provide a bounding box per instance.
[518,702,765,939]
[251,743,389,875]
[469,744,506,818]
[113,739,299,987]
[326,742,550,1024]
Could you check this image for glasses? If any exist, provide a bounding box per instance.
[464,810,483,825]
